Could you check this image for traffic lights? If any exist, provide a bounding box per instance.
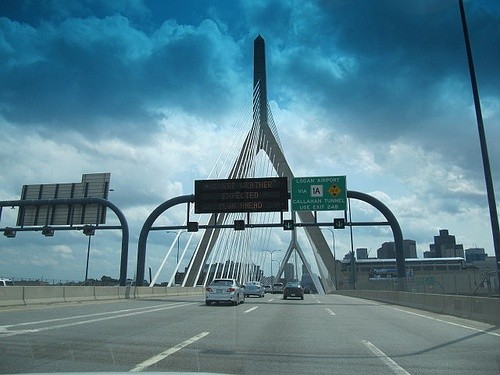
[284,220,294,230]
[334,218,345,229]
[187,222,199,232]
[235,220,244,230]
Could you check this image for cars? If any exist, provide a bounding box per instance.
[264,285,272,294]
[283,282,304,300]
[206,278,245,306]
[244,281,265,298]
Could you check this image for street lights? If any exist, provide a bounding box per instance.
[319,227,338,290]
[260,250,281,287]
[271,259,284,271]
[166,231,187,273]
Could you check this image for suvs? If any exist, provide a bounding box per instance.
[272,283,284,294]
[0,277,13,287]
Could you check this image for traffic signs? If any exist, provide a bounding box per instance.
[292,175,348,212]
